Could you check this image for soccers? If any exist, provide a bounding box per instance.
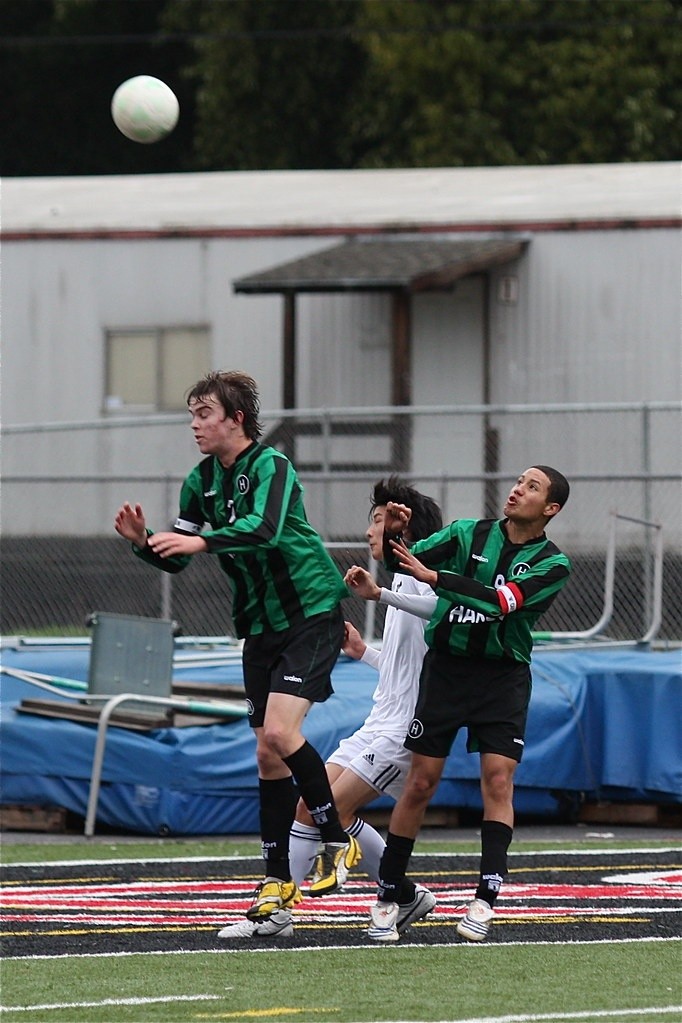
[112,74,181,143]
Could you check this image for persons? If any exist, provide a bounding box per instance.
[215,477,437,937]
[115,371,362,921]
[370,465,570,943]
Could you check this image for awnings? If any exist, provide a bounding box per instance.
[228,235,530,470]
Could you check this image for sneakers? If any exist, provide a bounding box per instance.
[307,832,361,897]
[394,882,441,934]
[217,905,296,937]
[454,897,493,941]
[246,880,302,924]
[368,898,400,941]
